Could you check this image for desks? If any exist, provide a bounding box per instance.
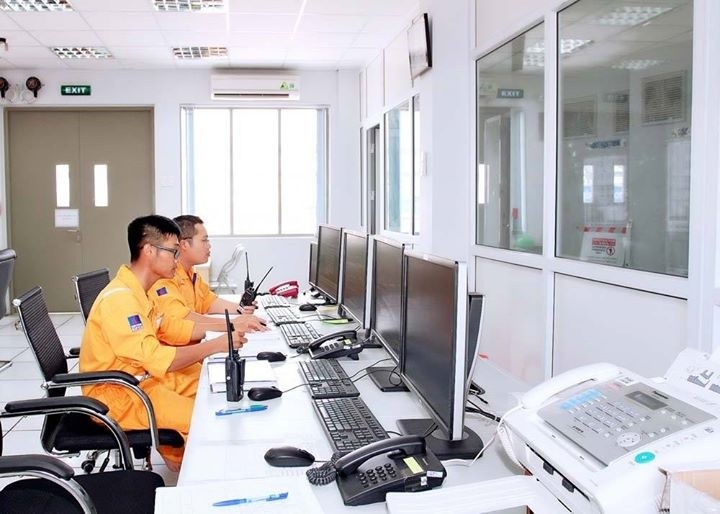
[178,292,528,514]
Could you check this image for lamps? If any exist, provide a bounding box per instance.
[26,76,42,97]
[0,77,9,97]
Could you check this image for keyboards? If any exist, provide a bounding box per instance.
[297,358,361,399]
[261,294,290,309]
[312,396,391,459]
[280,322,322,348]
[266,306,305,326]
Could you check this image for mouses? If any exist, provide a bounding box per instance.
[264,447,315,467]
[256,351,285,362]
[248,385,282,402]
[300,304,317,311]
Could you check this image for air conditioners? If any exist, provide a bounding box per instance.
[209,69,300,100]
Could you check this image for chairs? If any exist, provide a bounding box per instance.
[0,249,17,372]
[0,397,165,514]
[72,268,110,326]
[12,286,183,471]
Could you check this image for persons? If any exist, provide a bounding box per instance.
[146,215,267,383]
[79,214,265,472]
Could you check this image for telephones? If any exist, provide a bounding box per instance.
[518,362,620,408]
[308,330,363,360]
[333,435,446,506]
[268,281,300,299]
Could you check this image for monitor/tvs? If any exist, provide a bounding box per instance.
[365,235,411,393]
[395,249,484,461]
[405,12,432,79]
[306,242,318,294]
[313,224,344,307]
[340,227,383,347]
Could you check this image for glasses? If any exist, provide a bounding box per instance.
[140,244,179,259]
